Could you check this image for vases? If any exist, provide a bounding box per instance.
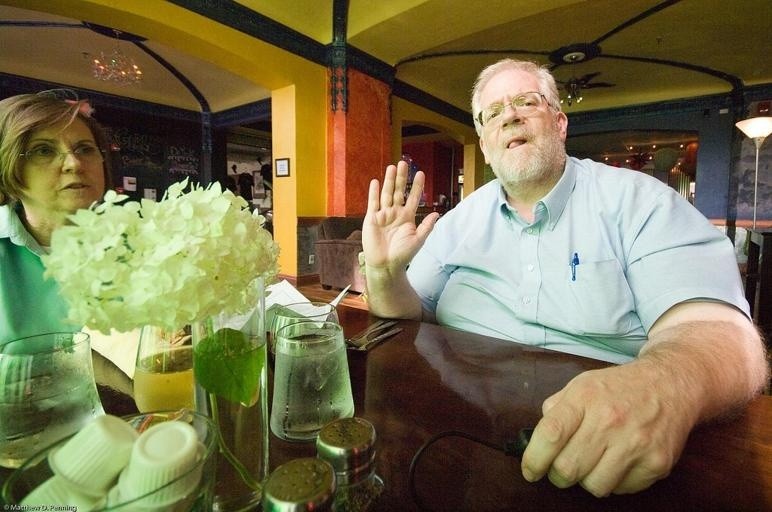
[189,285,270,512]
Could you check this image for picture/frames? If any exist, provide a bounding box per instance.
[275,158,290,177]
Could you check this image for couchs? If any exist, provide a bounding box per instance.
[312,216,423,294]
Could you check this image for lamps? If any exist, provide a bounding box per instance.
[734,115,772,231]
[560,87,583,107]
[80,29,144,90]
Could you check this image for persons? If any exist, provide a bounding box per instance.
[0,87,134,401]
[360,57,770,499]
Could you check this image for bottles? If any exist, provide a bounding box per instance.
[315,417,378,512]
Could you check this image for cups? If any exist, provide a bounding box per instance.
[269,321,356,444]
[0,331,107,471]
[270,300,339,371]
[133,324,195,425]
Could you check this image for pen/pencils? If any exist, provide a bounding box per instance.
[571,252,579,281]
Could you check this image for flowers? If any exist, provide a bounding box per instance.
[42,183,281,488]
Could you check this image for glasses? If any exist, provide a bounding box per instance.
[474,90,552,128]
[16,141,110,165]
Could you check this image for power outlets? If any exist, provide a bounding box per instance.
[308,255,315,263]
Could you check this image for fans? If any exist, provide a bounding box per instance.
[555,73,617,91]
